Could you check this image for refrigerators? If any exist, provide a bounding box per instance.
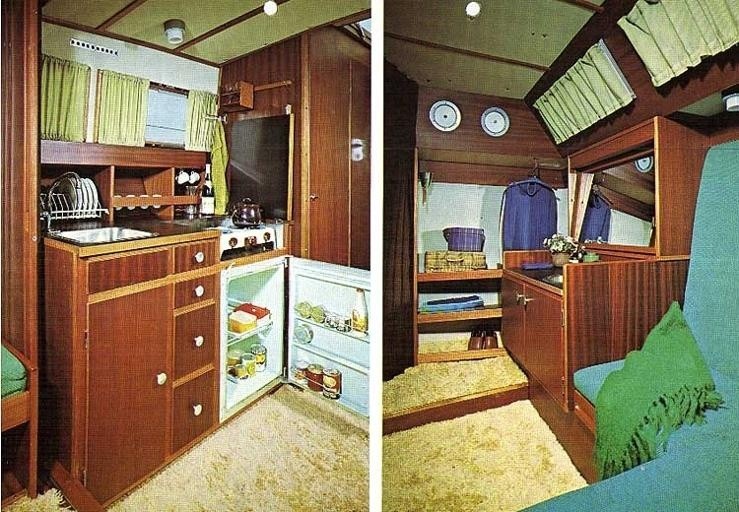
[219,256,372,422]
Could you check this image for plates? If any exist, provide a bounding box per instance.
[63,176,100,215]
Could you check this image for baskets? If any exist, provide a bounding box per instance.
[549,250,575,266]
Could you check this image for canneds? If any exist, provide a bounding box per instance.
[294,359,342,399]
[234,343,266,379]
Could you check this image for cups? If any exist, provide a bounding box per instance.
[175,170,200,184]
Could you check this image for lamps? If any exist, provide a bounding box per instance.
[262,1,278,17]
[721,92,738,111]
[464,2,483,20]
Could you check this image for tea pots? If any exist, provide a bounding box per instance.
[232,197,264,227]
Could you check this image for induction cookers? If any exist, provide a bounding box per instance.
[211,224,276,258]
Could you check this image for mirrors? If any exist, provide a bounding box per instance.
[573,155,655,250]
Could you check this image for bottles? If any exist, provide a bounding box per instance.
[201,163,216,217]
[350,287,368,339]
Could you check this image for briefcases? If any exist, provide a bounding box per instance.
[423,249,488,273]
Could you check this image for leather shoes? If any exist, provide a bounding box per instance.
[468,326,486,352]
[485,329,499,349]
